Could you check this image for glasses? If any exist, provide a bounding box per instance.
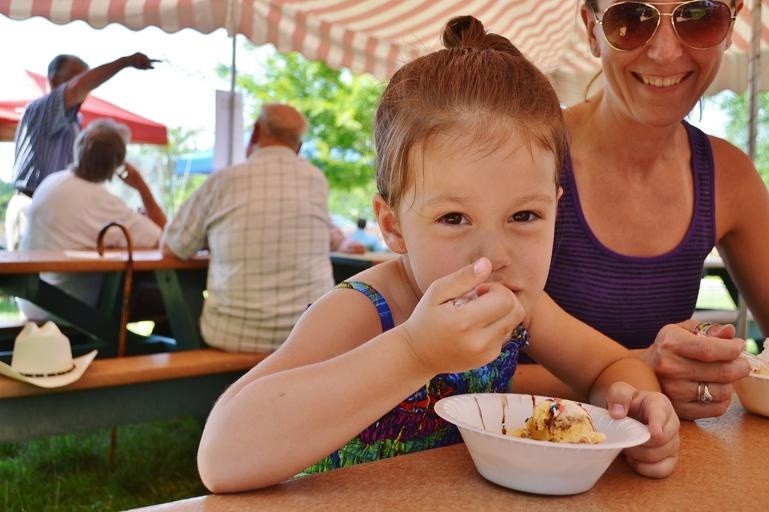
[585,0,736,54]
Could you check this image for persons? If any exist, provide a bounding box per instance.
[11,116,170,339]
[347,218,387,252]
[157,99,339,356]
[329,215,346,252]
[509,0,769,427]
[192,12,683,495]
[2,50,164,257]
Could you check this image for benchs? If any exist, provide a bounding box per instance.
[0,349,267,442]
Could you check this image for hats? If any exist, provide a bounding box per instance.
[0,320,100,390]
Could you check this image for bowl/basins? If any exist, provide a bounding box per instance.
[733,374,769,418]
[434,392,650,496]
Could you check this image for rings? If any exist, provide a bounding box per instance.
[695,383,714,404]
